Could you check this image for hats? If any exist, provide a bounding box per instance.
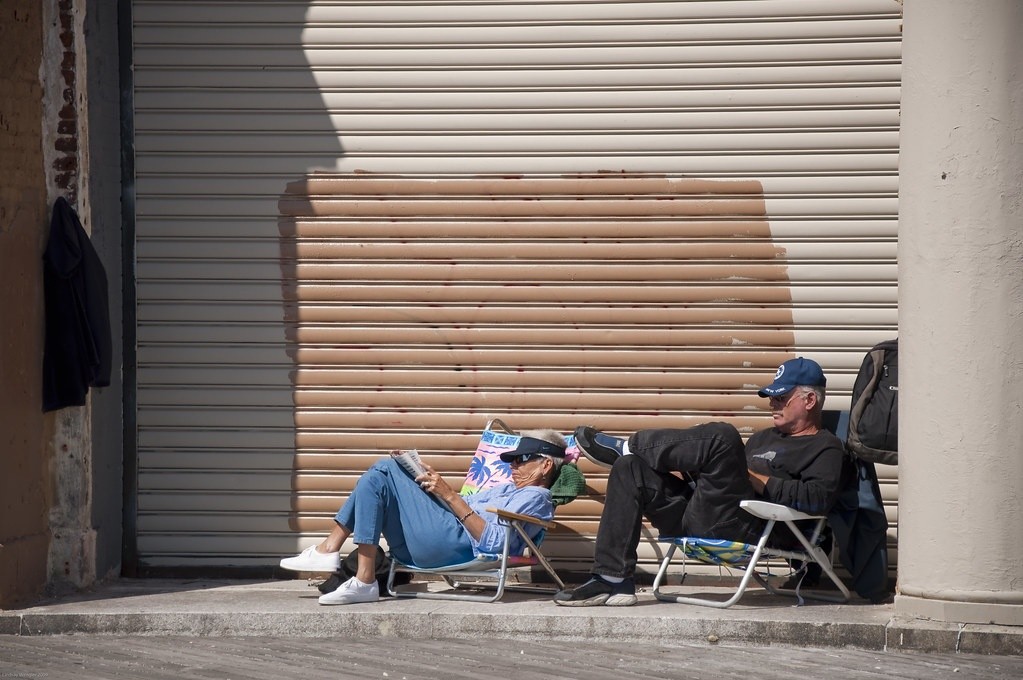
[758,357,826,398]
[500,437,565,463]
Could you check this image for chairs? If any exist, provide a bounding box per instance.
[651,411,890,609]
[386,418,582,604]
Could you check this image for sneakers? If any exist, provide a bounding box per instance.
[553,573,638,606]
[573,426,627,469]
[280,545,341,573]
[319,576,379,605]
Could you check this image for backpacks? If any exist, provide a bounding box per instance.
[846,337,898,466]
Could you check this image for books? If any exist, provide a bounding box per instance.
[388,449,426,483]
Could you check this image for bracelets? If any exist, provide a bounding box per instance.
[461,511,474,521]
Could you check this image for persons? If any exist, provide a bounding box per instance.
[280,429,566,604]
[554,356,846,607]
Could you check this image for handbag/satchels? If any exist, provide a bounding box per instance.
[318,545,414,596]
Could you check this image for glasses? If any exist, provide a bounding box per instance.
[768,387,809,402]
[512,453,547,464]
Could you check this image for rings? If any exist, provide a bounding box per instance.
[425,482,429,485]
